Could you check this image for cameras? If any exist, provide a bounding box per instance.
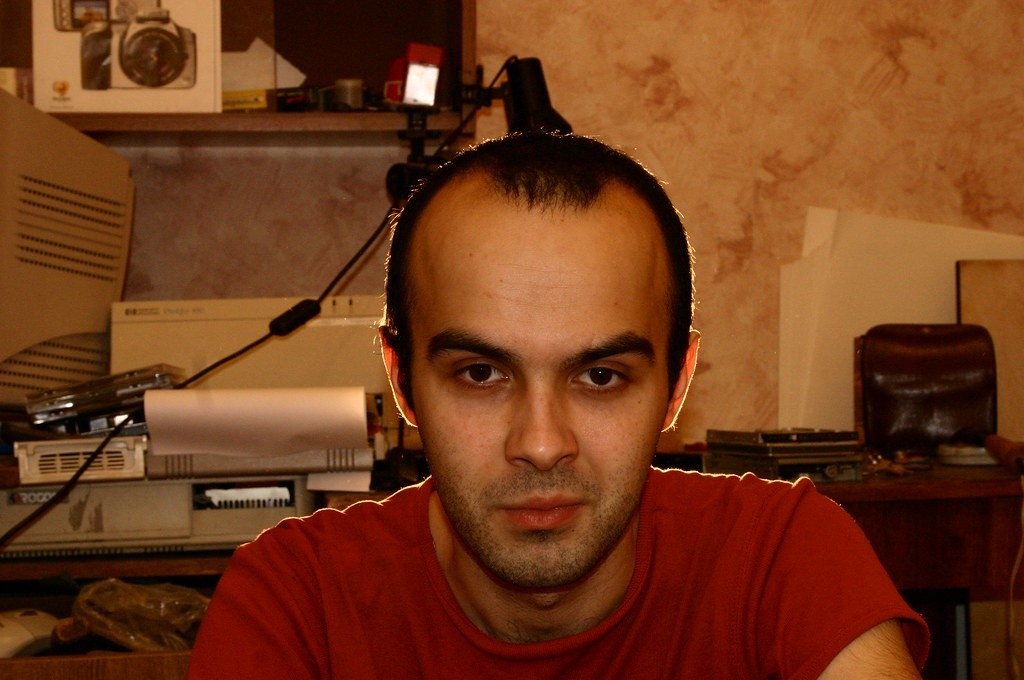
[51,0,161,32]
[78,6,198,90]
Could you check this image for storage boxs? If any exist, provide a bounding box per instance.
[105,295,403,458]
[0,0,280,114]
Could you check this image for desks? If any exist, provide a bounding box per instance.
[700,432,1023,680]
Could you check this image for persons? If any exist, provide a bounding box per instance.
[189,130,930,680]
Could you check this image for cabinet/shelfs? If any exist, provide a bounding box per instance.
[0,0,480,140]
